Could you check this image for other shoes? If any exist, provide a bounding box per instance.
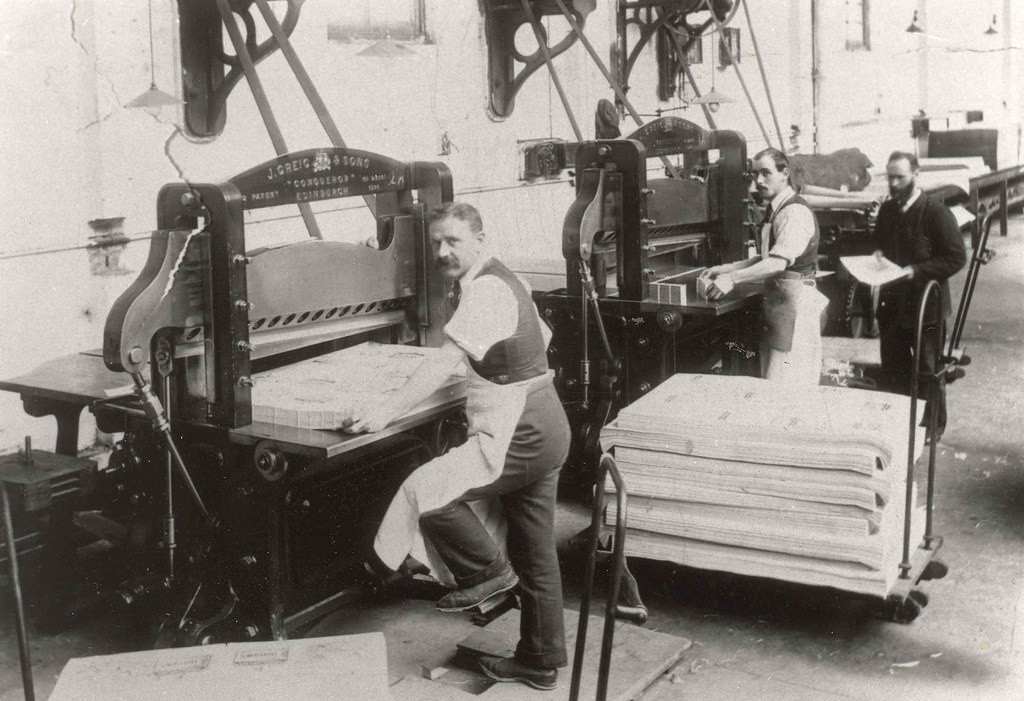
[925,433,941,445]
[434,560,520,613]
[477,656,559,690]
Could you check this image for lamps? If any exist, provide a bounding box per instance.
[983,24,997,34]
[716,27,740,72]
[355,0,420,58]
[123,0,188,115]
[906,9,923,32]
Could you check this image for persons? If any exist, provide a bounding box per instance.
[697,148,829,386]
[871,151,968,443]
[341,203,571,690]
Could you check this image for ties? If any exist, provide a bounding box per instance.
[897,200,906,215]
[762,202,773,227]
[449,280,460,308]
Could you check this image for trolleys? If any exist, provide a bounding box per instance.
[821,205,997,387]
[621,281,950,625]
